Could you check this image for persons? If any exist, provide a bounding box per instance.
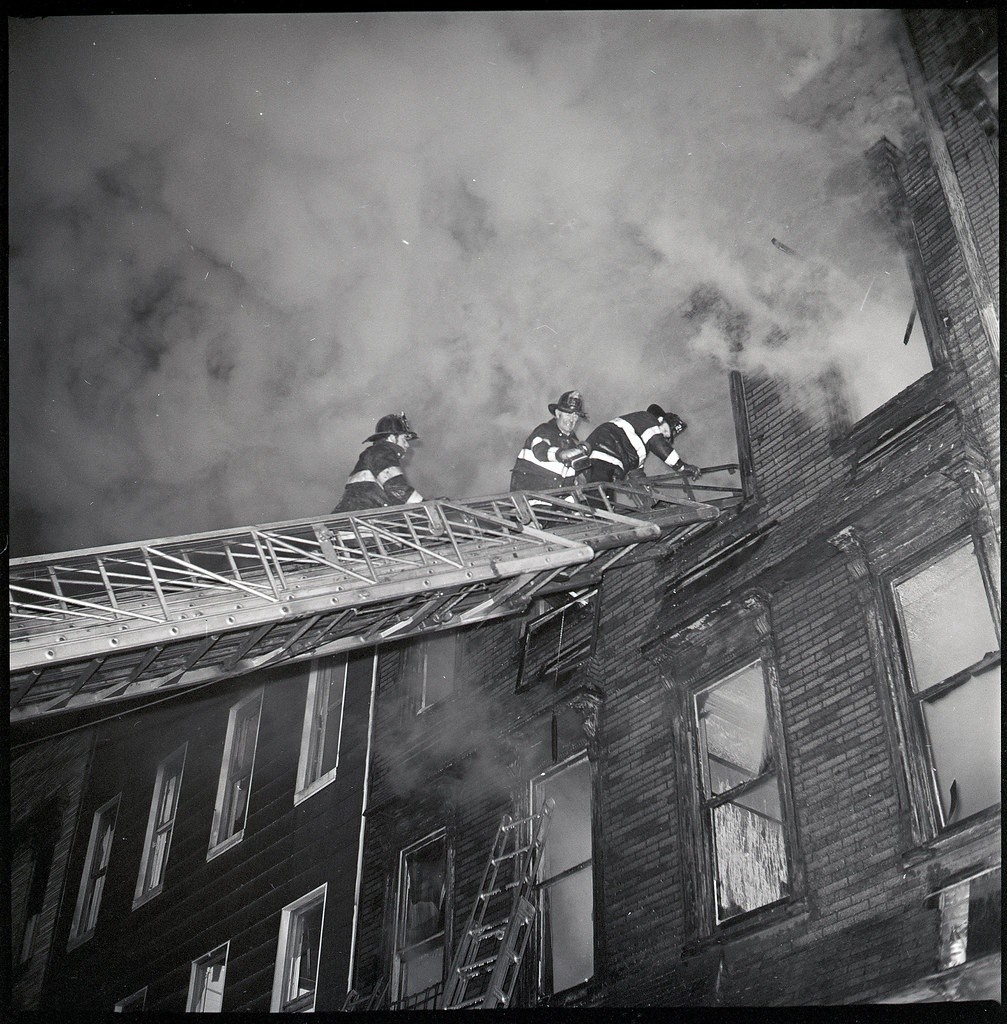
[326,414,450,558]
[585,404,701,512]
[509,391,592,583]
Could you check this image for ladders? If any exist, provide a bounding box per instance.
[7,459,743,726]
[435,797,557,1010]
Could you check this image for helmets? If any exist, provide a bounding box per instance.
[361,414,416,441]
[548,390,586,418]
[650,401,685,443]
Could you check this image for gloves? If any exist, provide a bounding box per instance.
[684,462,703,483]
